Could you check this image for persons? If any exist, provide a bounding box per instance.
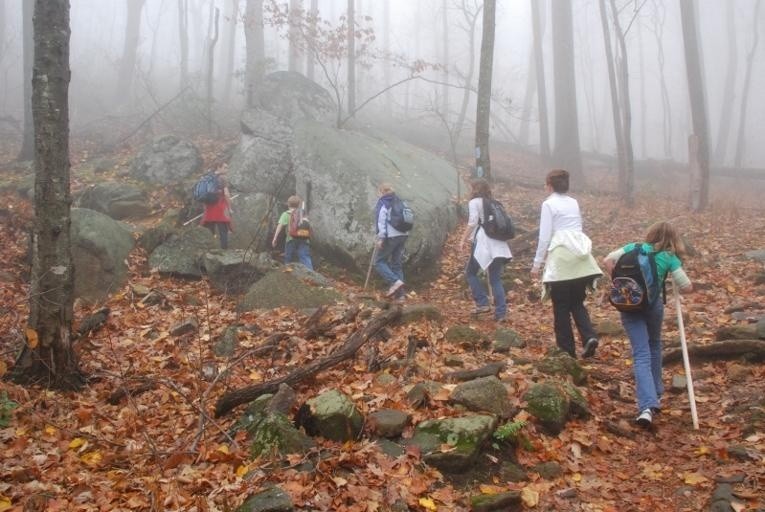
[460,180,514,322]
[271,195,314,271]
[530,169,606,359]
[373,183,409,301]
[199,160,234,250]
[602,220,693,427]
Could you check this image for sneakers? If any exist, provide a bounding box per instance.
[470,305,491,315]
[490,315,506,322]
[581,338,598,359]
[636,408,652,426]
[383,279,406,298]
[392,298,406,304]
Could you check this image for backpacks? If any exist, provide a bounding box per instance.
[388,195,413,232]
[609,242,660,312]
[193,173,223,205]
[481,195,516,241]
[289,207,312,238]
